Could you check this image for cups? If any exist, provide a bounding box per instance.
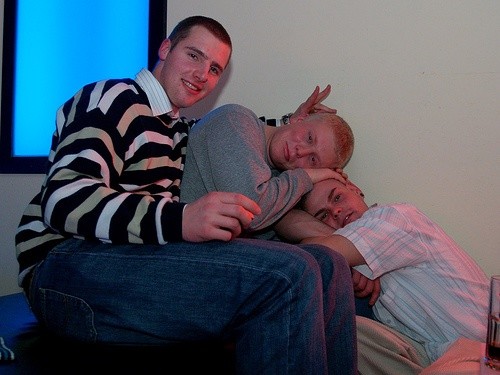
[484,275,500,371]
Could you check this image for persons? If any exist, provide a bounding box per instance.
[275,178,500,375]
[179,104,354,243]
[13,15,359,375]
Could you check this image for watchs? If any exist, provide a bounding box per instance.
[282,113,293,125]
